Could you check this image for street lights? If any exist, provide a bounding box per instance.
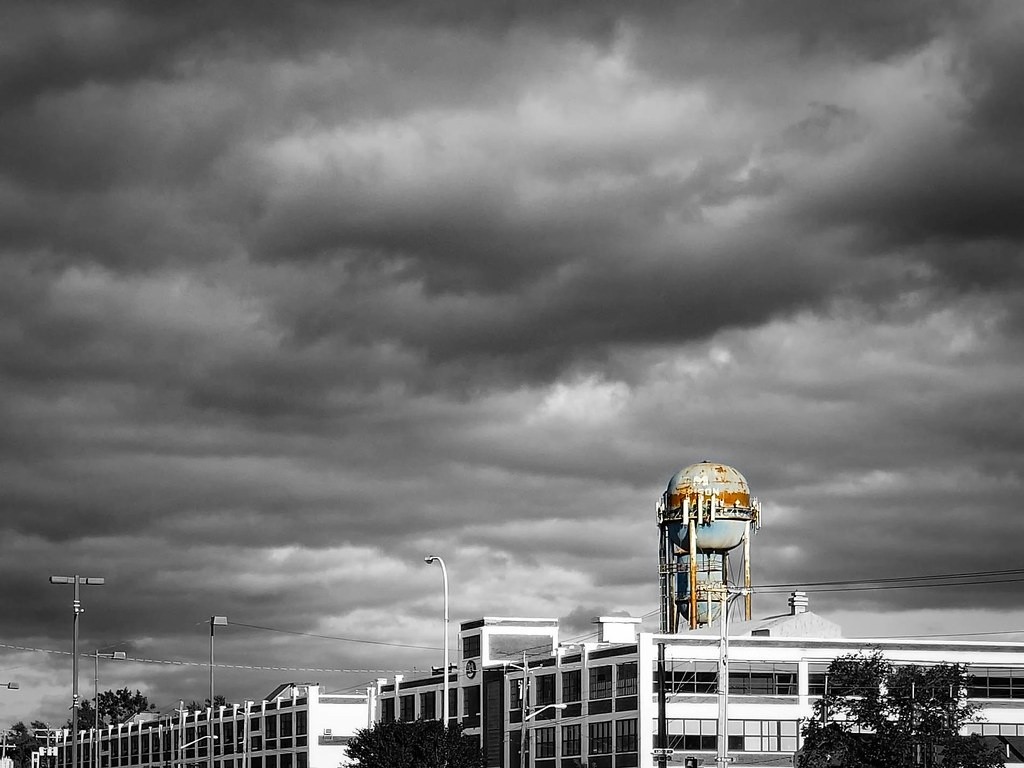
[48,574,105,768]
[422,554,450,768]
[95,652,128,768]
[520,703,568,768]
[177,735,218,768]
[206,616,229,767]
